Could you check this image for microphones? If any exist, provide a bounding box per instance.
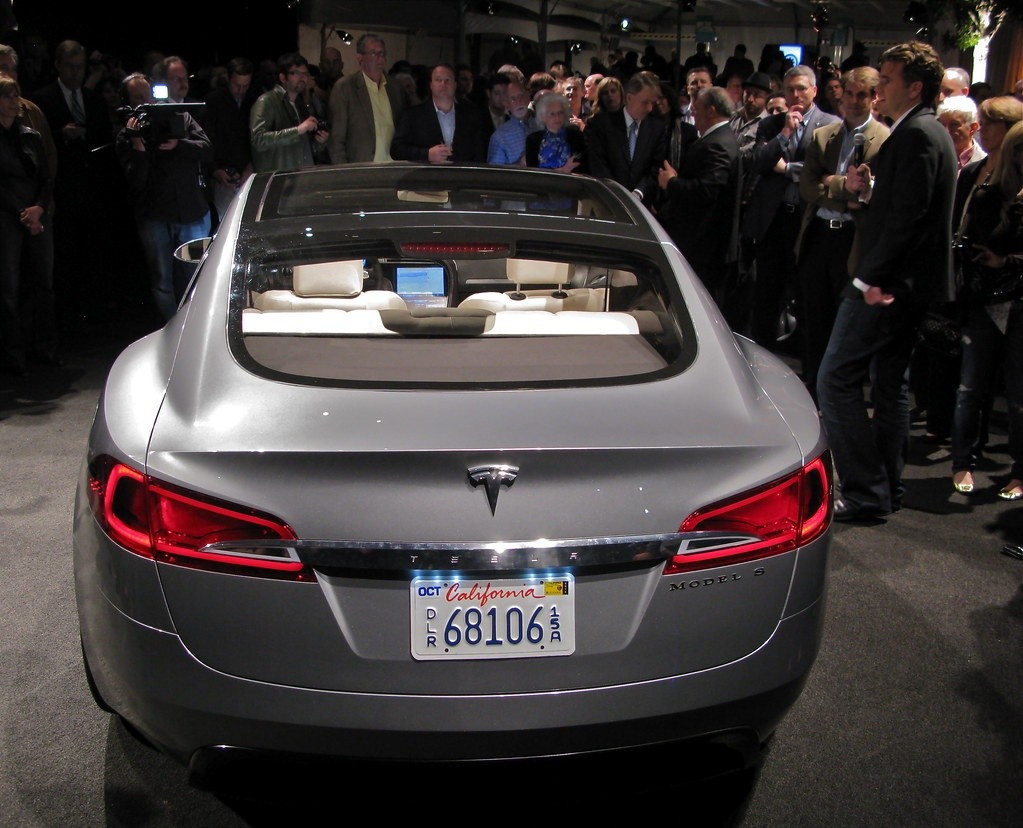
[853,133,865,197]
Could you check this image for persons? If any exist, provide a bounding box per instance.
[205,57,256,226]
[318,46,345,92]
[249,53,329,174]
[386,60,492,165]
[0,40,211,400]
[814,37,869,114]
[1002,540,1022,559]
[686,41,793,84]
[486,60,591,173]
[820,41,1022,523]
[589,44,684,84]
[730,65,891,411]
[329,33,412,165]
[584,65,740,300]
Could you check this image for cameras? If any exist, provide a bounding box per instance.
[304,104,332,134]
[227,172,242,188]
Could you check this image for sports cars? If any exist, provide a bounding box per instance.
[70,158,841,806]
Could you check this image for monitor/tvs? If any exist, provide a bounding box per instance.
[778,45,803,67]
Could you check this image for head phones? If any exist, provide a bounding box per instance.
[117,72,155,106]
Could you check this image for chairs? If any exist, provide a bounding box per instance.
[251,257,407,313]
[456,254,609,311]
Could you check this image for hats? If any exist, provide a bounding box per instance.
[741,72,774,95]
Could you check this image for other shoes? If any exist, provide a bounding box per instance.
[998,480,1023,500]
[1002,543,1023,560]
[922,432,944,443]
[954,470,974,493]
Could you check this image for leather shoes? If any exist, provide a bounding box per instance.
[836,484,842,492]
[832,497,892,518]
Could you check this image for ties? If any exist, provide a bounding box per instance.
[629,121,637,161]
[71,90,85,139]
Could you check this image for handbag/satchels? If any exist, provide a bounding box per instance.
[949,183,1023,305]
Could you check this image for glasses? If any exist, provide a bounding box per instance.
[360,50,386,58]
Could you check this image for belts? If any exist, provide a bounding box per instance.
[814,216,853,229]
